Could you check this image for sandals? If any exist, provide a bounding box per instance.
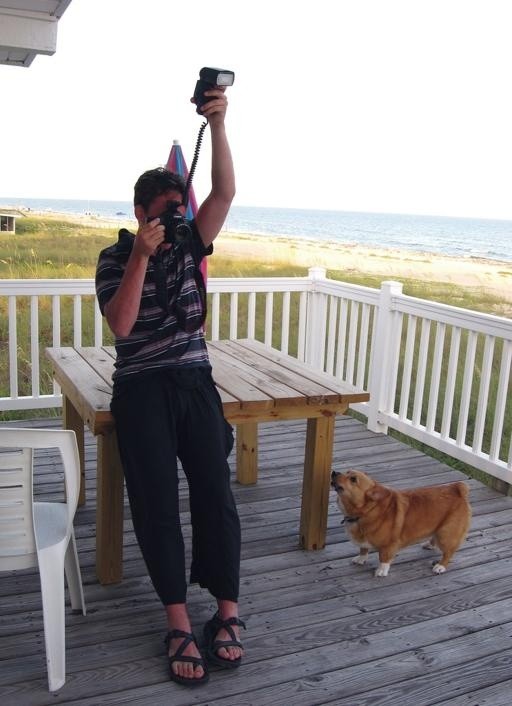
[164,629,210,687]
[204,610,247,669]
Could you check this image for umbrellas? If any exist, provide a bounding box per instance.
[162,139,211,345]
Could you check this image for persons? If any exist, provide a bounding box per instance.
[93,83,248,688]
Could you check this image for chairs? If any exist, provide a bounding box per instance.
[1,428,86,693]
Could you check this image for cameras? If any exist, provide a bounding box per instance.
[147,200,192,244]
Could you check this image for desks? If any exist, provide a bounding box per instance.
[42,337,372,586]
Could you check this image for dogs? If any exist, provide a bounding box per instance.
[330,468,473,579]
